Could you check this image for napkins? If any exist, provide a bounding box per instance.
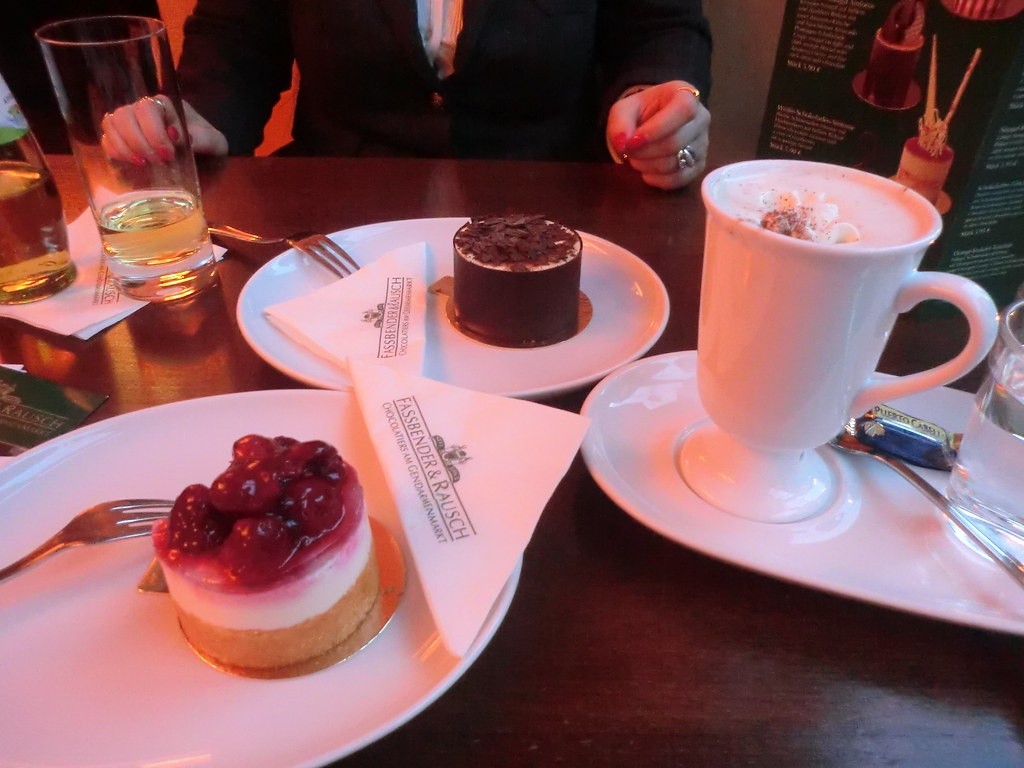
[0,185,228,340]
[349,358,595,662]
[264,240,426,374]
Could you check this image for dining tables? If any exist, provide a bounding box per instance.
[0,156,1024,768]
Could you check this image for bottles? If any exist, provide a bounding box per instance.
[0,74,78,304]
[947,298,1024,540]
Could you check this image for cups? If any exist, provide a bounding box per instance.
[864,26,924,110]
[673,159,998,521]
[901,139,953,204]
[36,15,215,304]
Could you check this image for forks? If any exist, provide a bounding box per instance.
[0,498,175,583]
[207,223,361,280]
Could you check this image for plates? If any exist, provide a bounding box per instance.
[886,176,951,215]
[853,71,923,109]
[580,350,1024,634]
[236,217,670,397]
[0,389,523,768]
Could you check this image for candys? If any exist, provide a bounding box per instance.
[841,404,964,471]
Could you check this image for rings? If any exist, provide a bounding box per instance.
[99,133,106,145]
[102,112,114,119]
[678,145,696,169]
[139,96,168,119]
[676,86,700,100]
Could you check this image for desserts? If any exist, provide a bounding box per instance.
[151,434,378,670]
[450,212,583,347]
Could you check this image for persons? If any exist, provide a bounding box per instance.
[99,0,714,190]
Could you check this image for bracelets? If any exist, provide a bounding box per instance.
[624,86,644,98]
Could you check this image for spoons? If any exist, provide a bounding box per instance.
[828,418,1024,589]
